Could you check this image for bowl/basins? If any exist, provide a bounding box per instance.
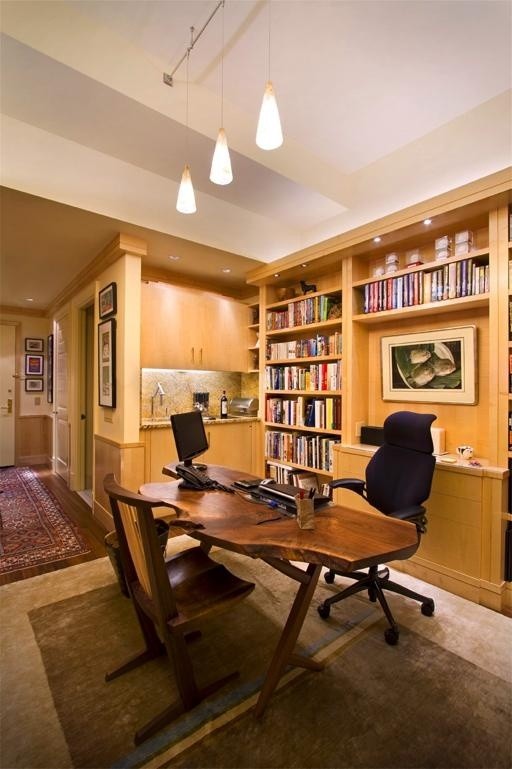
[275,287,296,302]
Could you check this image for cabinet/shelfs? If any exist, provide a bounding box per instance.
[245,167,511,609]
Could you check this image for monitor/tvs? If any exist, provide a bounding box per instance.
[170,410,208,470]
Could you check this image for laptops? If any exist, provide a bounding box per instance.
[258,484,331,503]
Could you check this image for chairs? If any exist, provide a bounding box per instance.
[108,474,256,747]
[311,410,437,647]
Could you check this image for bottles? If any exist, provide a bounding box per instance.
[221,390,228,419]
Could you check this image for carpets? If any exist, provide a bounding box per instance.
[26,546,512,769]
[0,465,96,575]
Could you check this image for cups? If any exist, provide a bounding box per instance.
[456,445,474,460]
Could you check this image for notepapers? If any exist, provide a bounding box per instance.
[441,458,457,463]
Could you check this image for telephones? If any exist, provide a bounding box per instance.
[176,464,213,488]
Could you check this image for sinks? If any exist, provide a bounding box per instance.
[143,417,171,426]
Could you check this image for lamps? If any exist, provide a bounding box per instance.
[175,51,197,216]
[208,2,233,185]
[255,0,283,150]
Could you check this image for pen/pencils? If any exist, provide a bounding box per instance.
[299,488,316,500]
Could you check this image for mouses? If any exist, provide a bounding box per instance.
[260,479,276,485]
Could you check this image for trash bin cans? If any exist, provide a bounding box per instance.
[104,519,169,599]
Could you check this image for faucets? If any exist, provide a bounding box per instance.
[152,382,165,414]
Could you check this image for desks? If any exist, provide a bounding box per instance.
[138,461,420,720]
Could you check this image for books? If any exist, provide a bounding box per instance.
[509,352,512,393]
[265,295,341,502]
[509,410,512,452]
[231,477,331,514]
[363,257,490,313]
[508,302,512,340]
[508,259,512,293]
[508,212,512,241]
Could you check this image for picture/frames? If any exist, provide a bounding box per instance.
[98,319,115,409]
[381,324,480,406]
[98,280,116,319]
[25,378,44,392]
[25,337,44,353]
[47,335,53,403]
[25,354,44,376]
